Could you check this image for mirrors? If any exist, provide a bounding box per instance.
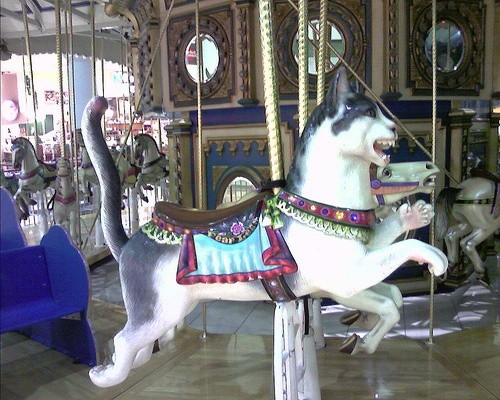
[221,176,257,203]
[424,18,466,73]
[291,18,346,76]
[184,33,220,85]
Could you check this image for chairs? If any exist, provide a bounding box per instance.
[0,186,100,367]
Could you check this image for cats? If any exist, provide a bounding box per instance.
[80,66,449,388]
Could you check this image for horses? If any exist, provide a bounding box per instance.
[0,128,170,246]
[159,157,440,357]
[434,170,500,289]
